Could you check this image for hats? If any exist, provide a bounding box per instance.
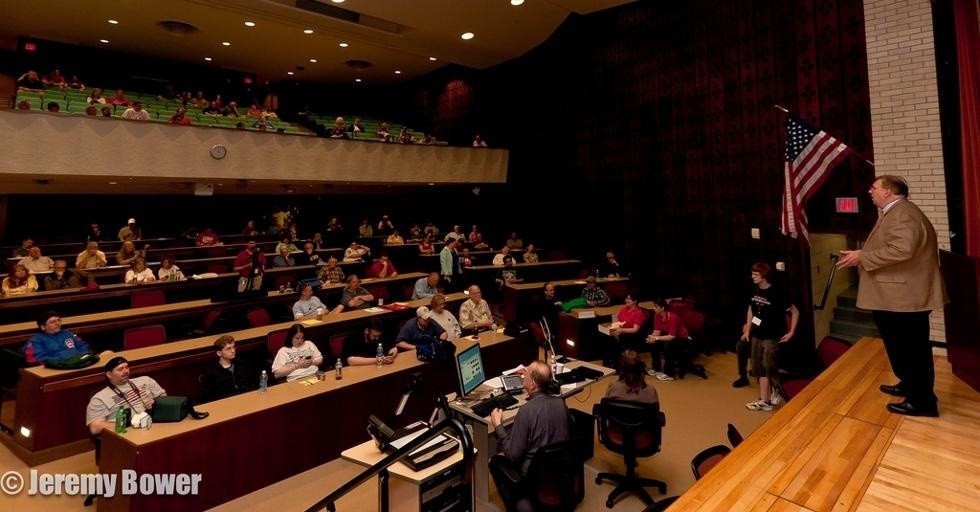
[583,276,596,283]
[36,309,61,326]
[416,305,432,321]
[98,350,129,372]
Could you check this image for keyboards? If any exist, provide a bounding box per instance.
[556,372,586,386]
[470,392,519,418]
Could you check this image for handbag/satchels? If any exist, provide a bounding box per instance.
[413,333,457,365]
[151,394,209,424]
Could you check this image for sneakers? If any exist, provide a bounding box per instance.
[655,373,674,381]
[768,383,782,406]
[647,368,659,376]
[744,398,773,411]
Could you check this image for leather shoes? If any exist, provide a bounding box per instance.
[732,377,750,388]
[879,384,907,397]
[885,396,939,417]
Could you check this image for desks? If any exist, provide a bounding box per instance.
[1,290,473,469]
[457,259,583,304]
[1,269,430,406]
[447,353,617,511]
[418,247,541,284]
[376,238,488,269]
[500,277,630,323]
[94,328,515,512]
[0,258,377,325]
[559,296,683,362]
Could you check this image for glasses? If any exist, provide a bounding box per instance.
[607,257,615,259]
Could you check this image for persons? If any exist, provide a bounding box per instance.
[67,72,85,92]
[377,216,394,227]
[459,248,475,267]
[24,312,87,360]
[86,89,105,104]
[597,252,622,280]
[14,238,35,257]
[296,242,320,267]
[45,69,64,91]
[241,221,266,236]
[107,87,129,106]
[445,226,466,241]
[497,256,524,291]
[533,283,568,339]
[275,235,298,256]
[425,220,440,242]
[492,248,515,266]
[471,134,486,147]
[397,307,448,350]
[507,233,522,248]
[271,323,326,380]
[522,244,536,261]
[413,273,444,299]
[121,101,150,121]
[370,254,398,278]
[419,236,435,254]
[644,296,689,381]
[615,293,643,350]
[75,242,107,269]
[836,174,941,419]
[343,243,370,261]
[292,284,344,321]
[410,222,421,238]
[309,231,323,248]
[82,105,98,116]
[201,335,260,398]
[118,219,141,240]
[387,230,405,243]
[341,276,374,311]
[87,222,101,240]
[99,103,113,115]
[197,228,219,246]
[426,293,461,341]
[488,361,576,512]
[86,356,166,441]
[742,261,800,414]
[3,264,38,296]
[359,218,372,239]
[15,100,32,109]
[468,225,481,241]
[580,276,609,308]
[21,71,43,93]
[175,85,449,145]
[126,257,154,284]
[459,285,496,336]
[327,217,343,230]
[438,239,463,289]
[17,249,54,273]
[47,260,85,289]
[168,107,190,123]
[160,254,183,279]
[603,354,660,442]
[47,99,61,114]
[318,258,344,285]
[236,239,263,291]
[117,242,144,265]
[343,317,398,366]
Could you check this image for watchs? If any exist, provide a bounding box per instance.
[655,335,658,340]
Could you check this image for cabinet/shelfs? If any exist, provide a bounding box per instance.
[340,418,477,512]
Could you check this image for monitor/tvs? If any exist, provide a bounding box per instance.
[501,374,525,391]
[455,342,485,398]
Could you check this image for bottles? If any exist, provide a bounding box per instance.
[286,283,292,294]
[550,355,556,375]
[335,358,342,379]
[115,405,126,433]
[170,269,175,281]
[377,291,384,308]
[133,271,138,284]
[176,267,180,281]
[596,268,600,278]
[259,370,268,392]
[280,286,284,295]
[376,343,384,365]
[317,305,325,321]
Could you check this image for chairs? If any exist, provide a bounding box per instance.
[306,115,449,146]
[486,407,596,511]
[624,307,655,354]
[591,397,668,512]
[266,327,291,384]
[124,324,167,350]
[205,261,230,275]
[528,320,552,365]
[328,332,349,368]
[779,375,812,403]
[398,318,410,332]
[691,444,731,481]
[669,300,695,313]
[83,433,102,506]
[726,422,744,448]
[815,335,854,368]
[244,306,293,328]
[272,271,300,290]
[401,284,416,301]
[366,283,404,307]
[13,85,299,133]
[353,261,378,278]
[20,340,47,368]
[130,286,167,309]
[197,373,206,383]
[674,308,709,379]
[603,281,631,304]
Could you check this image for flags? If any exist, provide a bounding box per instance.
[781,109,855,243]
